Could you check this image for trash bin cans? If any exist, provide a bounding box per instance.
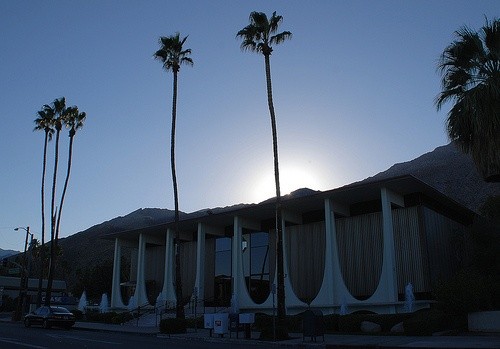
[213,312,230,338]
[302,309,325,342]
[204,313,215,337]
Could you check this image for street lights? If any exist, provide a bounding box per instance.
[14,228,33,290]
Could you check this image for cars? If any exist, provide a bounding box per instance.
[24,305,76,329]
